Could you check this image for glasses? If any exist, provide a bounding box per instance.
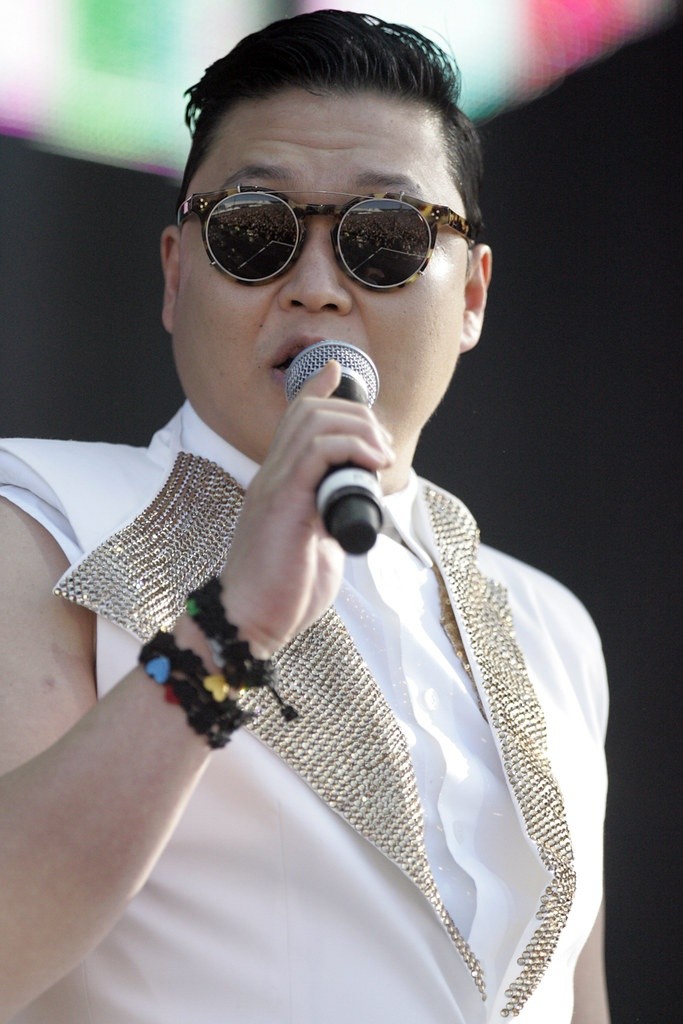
[178,186,476,293]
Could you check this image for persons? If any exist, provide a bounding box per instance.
[0,7,611,1024]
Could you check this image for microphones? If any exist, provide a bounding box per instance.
[281,339,385,555]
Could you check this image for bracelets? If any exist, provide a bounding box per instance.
[137,574,278,752]
